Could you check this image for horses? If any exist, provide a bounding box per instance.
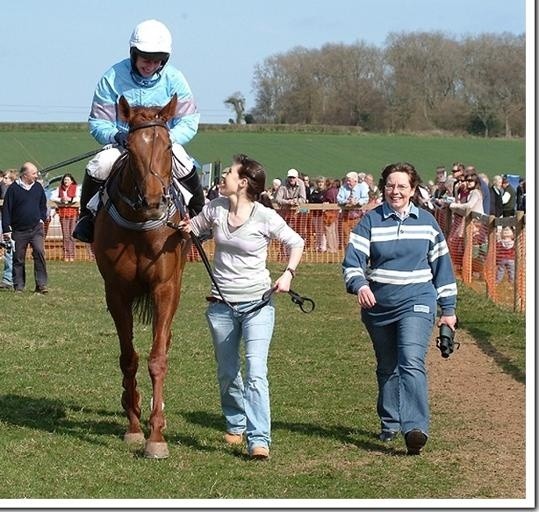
[91,92,189,460]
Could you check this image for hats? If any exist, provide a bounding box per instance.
[287,169,298,178]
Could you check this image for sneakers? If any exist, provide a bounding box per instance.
[251,446,270,459]
[225,431,243,444]
[36,286,48,293]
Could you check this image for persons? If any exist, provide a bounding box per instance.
[179,154,305,459]
[342,163,458,454]
[80,20,205,215]
[0,160,49,295]
[267,167,525,284]
[51,173,82,262]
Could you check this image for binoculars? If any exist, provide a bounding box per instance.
[437,313,458,358]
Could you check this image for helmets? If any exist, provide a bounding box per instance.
[129,19,173,66]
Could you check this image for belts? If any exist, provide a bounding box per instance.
[206,295,250,305]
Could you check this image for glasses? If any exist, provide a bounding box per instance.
[465,179,474,183]
[383,182,412,191]
[451,169,461,174]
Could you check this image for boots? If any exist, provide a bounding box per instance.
[177,166,213,240]
[72,168,108,242]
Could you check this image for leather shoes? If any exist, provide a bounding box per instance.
[406,428,427,455]
[379,431,398,442]
